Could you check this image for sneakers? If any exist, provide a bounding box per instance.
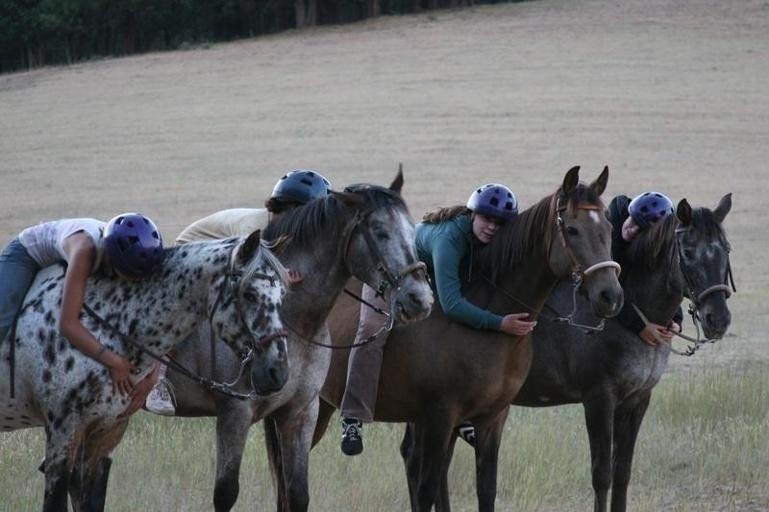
[459,426,475,448]
[145,382,175,415]
[341,417,363,455]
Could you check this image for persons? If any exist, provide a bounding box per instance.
[604,191,684,346]
[145,169,333,415]
[340,184,538,456]
[0,213,164,415]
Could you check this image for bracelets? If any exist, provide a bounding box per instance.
[93,345,107,357]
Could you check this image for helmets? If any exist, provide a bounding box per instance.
[628,191,674,230]
[271,169,332,203]
[467,184,518,218]
[104,212,163,278]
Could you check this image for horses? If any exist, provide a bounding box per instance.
[0,227,309,511]
[443,193,733,512]
[262,163,626,512]
[139,163,435,511]
